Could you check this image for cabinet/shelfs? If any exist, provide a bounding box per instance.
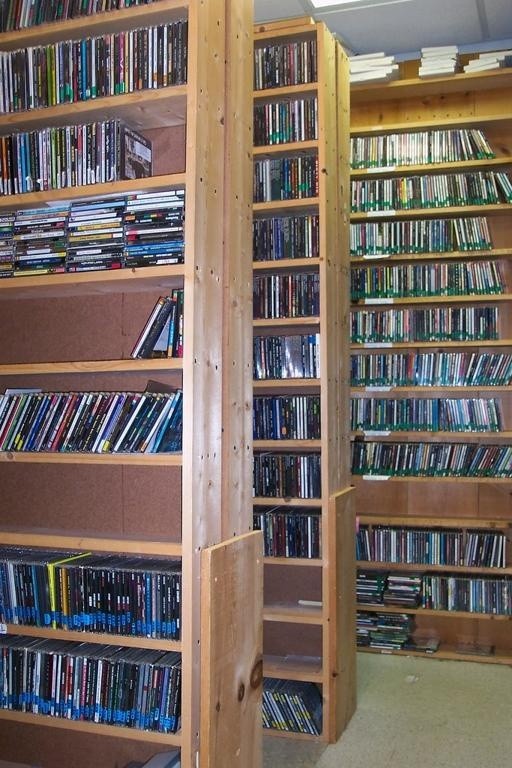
[348,43,512,663]
[4,1,265,768]
[252,17,358,746]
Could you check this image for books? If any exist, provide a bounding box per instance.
[356,526,511,656]
[350,129,511,479]
[349,44,510,88]
[1,546,182,740]
[253,40,323,560]
[131,287,184,359]
[2,3,190,277]
[0,389,183,455]
[260,686,323,735]
